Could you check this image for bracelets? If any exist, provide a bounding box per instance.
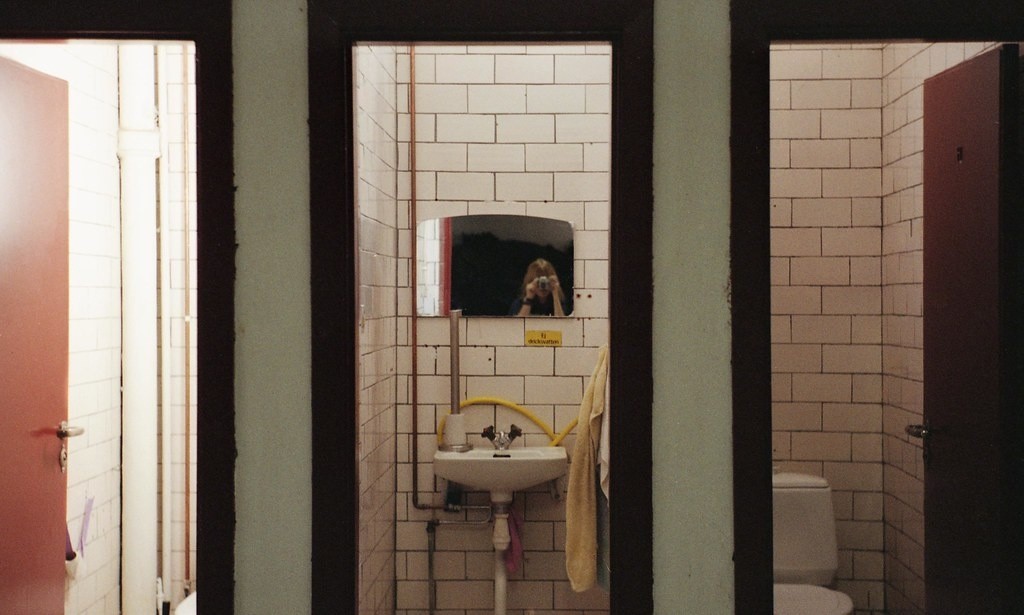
[522,298,532,305]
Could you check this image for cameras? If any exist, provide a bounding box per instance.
[536,276,552,290]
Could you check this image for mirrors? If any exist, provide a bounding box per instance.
[415,213,575,319]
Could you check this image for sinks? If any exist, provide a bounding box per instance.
[434,445,569,503]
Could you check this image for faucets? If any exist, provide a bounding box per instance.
[481,423,523,450]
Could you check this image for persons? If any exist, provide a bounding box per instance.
[507,259,568,317]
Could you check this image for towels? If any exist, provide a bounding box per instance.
[565,343,609,592]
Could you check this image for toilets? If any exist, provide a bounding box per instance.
[772,472,854,613]
[173,591,196,614]
